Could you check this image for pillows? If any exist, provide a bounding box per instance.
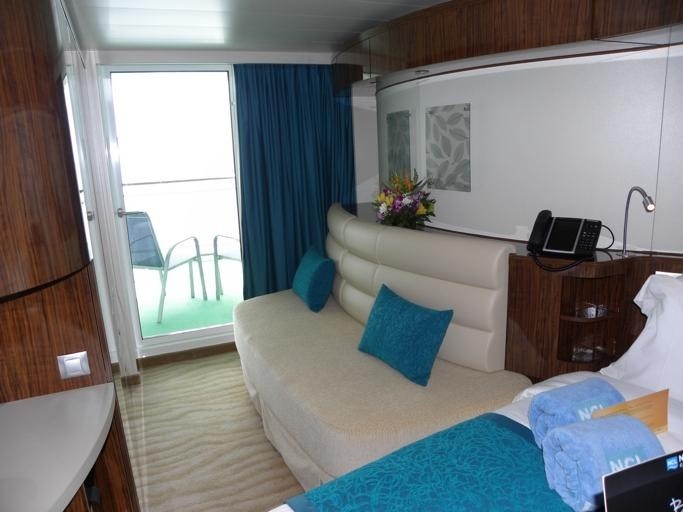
[291,243,335,313]
[357,283,453,386]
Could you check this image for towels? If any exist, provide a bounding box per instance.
[526,415,666,512]
[526,379,625,450]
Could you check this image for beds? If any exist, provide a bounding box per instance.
[266,275,682,512]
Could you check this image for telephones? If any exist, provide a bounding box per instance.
[527,210,602,261]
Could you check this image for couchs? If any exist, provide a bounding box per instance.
[234,202,532,491]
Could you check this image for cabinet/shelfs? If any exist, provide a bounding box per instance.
[504,250,682,379]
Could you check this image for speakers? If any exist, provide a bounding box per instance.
[332,63,363,97]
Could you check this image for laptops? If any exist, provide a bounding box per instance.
[602,450,683,512]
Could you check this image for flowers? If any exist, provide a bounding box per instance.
[370,167,436,229]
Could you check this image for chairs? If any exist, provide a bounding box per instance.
[213,235,241,300]
[124,211,207,324]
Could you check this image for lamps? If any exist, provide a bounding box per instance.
[623,186,655,253]
